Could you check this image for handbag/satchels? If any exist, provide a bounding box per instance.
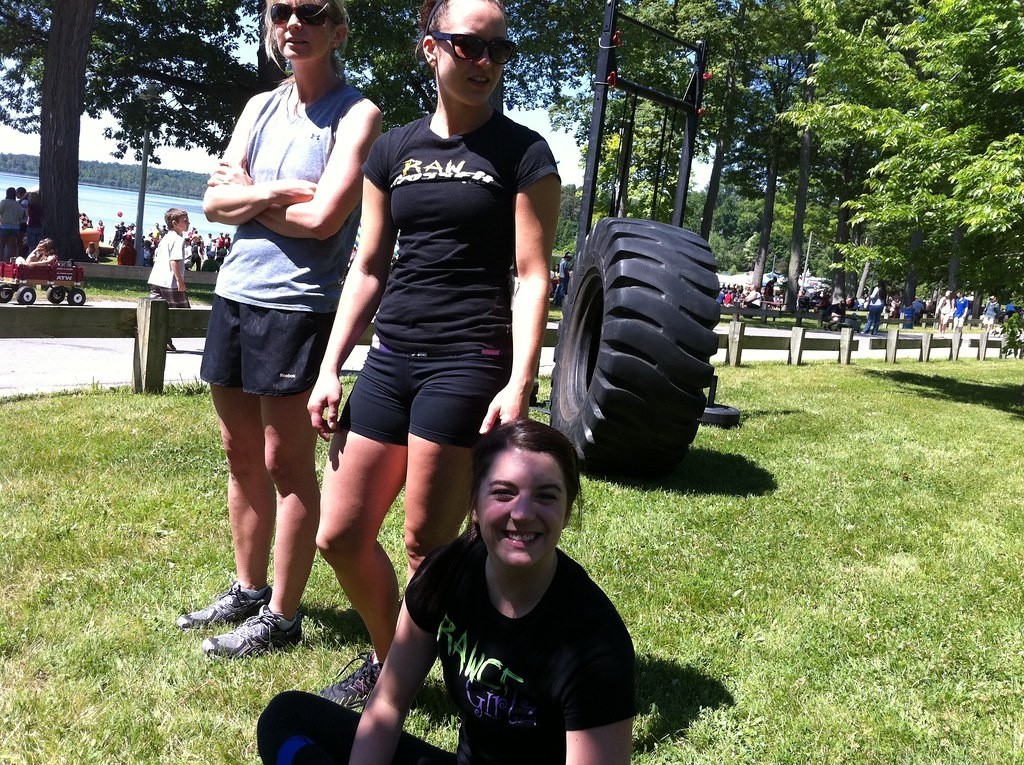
[985,309,987,313]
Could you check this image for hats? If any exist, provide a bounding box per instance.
[566,254,573,259]
[946,290,952,296]
[917,299,922,301]
[207,251,216,258]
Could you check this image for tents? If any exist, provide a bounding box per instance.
[716,270,821,287]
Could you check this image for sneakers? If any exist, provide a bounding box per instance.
[320,650,383,709]
[202,604,302,661]
[177,581,272,630]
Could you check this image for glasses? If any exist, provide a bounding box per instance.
[429,30,516,65]
[270,3,338,26]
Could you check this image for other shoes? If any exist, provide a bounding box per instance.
[166,343,176,351]
[824,325,832,331]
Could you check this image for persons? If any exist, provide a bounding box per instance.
[716,278,1023,334]
[0,186,231,272]
[306,0,561,709]
[257,417,638,765]
[549,250,577,306]
[178,0,381,656]
[146,207,192,351]
